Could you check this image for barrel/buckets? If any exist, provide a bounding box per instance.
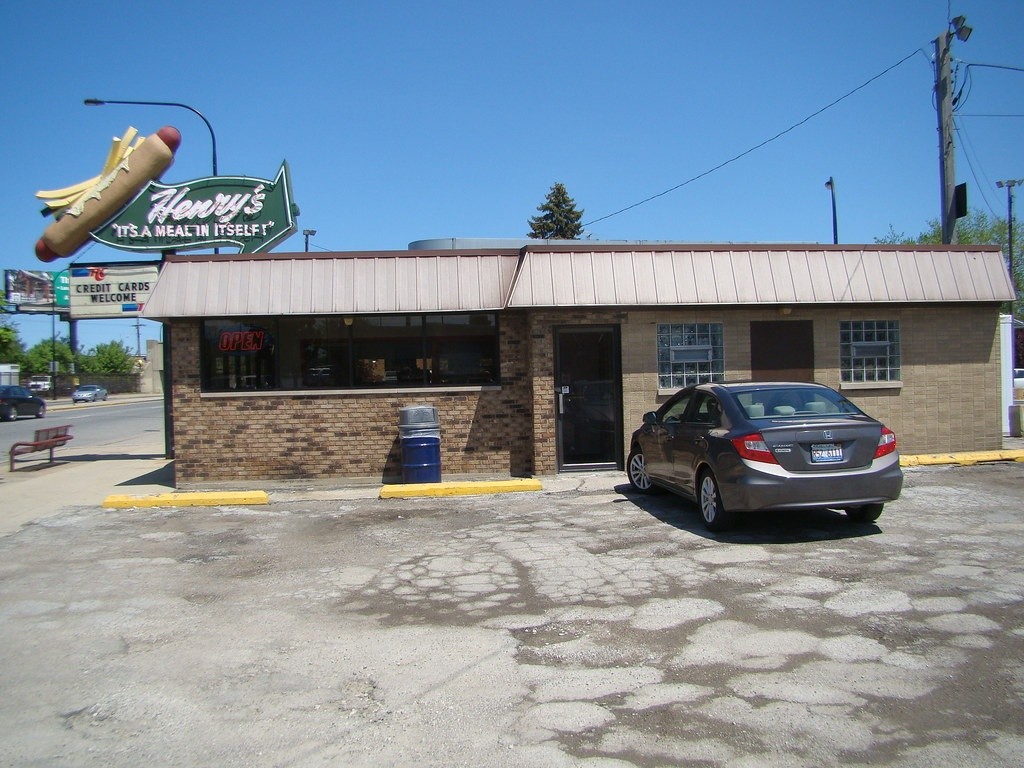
[398,406,442,482]
[1013,388,1024,404]
[1009,405,1024,436]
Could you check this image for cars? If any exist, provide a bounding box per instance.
[383,370,401,385]
[238,375,266,389]
[628,381,902,534]
[72,385,108,403]
[0,384,47,421]
[306,367,331,386]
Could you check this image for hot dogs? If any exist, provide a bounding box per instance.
[34,124,181,261]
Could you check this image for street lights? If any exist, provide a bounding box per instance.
[825,176,838,245]
[303,229,317,252]
[83,97,220,254]
[995,179,1024,388]
[930,15,972,246]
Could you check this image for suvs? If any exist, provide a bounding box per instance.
[28,375,54,395]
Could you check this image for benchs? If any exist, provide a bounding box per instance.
[8,423,74,473]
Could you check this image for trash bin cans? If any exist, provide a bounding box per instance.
[398,407,441,483]
[1008,406,1024,437]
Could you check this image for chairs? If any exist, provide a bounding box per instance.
[706,399,721,422]
[744,401,827,418]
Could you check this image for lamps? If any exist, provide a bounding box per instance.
[344,317,354,327]
[146,324,164,371]
[303,230,316,252]
